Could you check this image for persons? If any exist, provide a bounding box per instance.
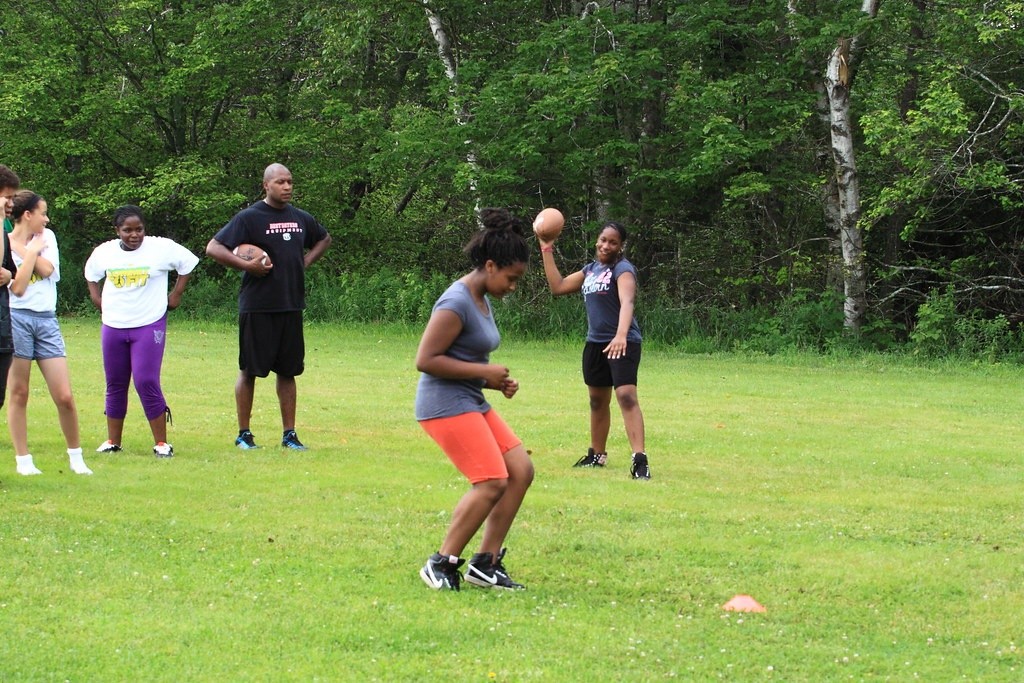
[414,208,535,594]
[6,189,94,477]
[0,163,20,411]
[84,206,200,458]
[531,221,651,482]
[205,162,333,451]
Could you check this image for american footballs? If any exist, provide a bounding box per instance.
[534,208,564,240]
[231,244,271,267]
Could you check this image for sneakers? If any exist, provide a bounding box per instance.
[95,439,174,457]
[236,428,307,451]
[572,448,652,481]
[419,548,526,596]
